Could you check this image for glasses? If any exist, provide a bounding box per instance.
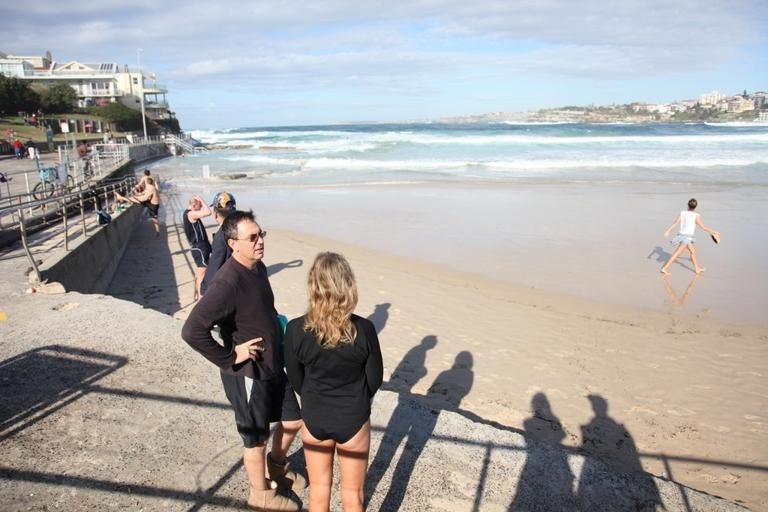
[233,230,266,242]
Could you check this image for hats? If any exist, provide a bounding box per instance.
[209,192,236,210]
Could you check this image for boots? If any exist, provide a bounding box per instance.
[246,479,300,512]
[266,451,306,491]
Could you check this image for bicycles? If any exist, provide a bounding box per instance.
[32,165,75,202]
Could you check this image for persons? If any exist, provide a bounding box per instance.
[660,199,720,274]
[662,272,701,307]
[181,208,309,511]
[283,251,384,512]
[0,106,117,183]
[182,193,212,303]
[199,191,237,297]
[113,178,161,234]
[132,170,159,195]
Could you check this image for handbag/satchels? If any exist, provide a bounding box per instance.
[97,209,111,225]
